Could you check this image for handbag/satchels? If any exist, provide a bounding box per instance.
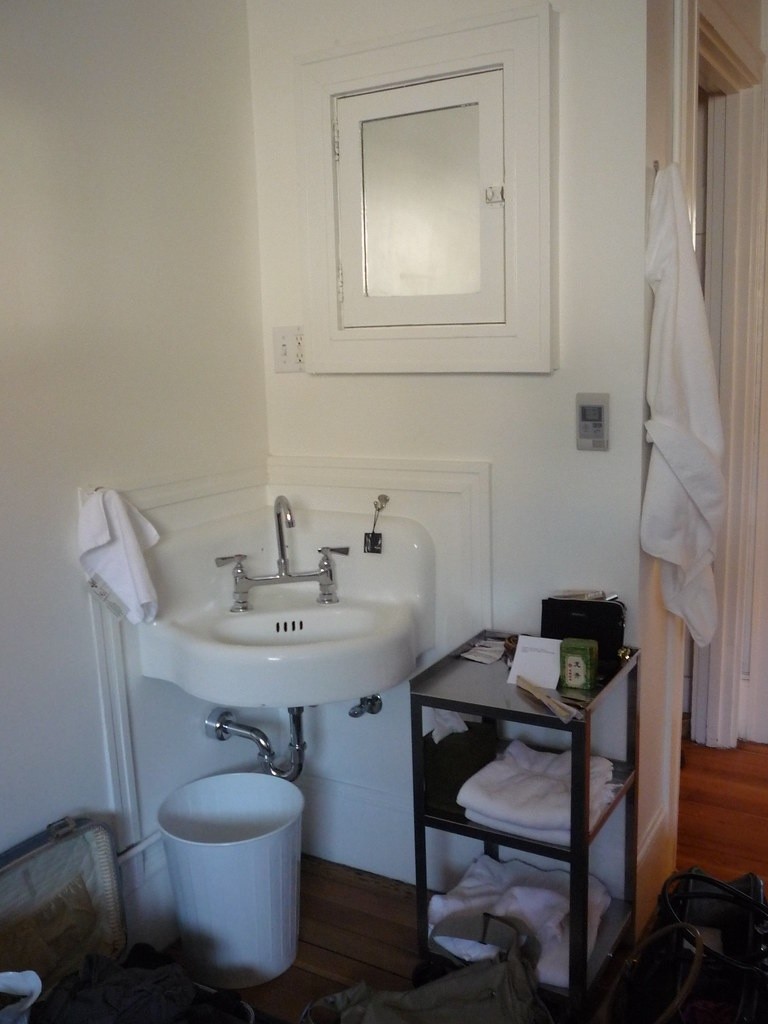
[604,873,768,1024]
[300,914,552,1024]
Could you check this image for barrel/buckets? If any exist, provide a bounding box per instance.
[157,773,305,988]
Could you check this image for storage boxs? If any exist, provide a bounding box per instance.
[560,638,598,689]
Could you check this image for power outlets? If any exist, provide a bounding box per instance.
[272,324,307,373]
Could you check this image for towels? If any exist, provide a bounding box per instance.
[640,160,728,650]
[427,853,612,990]
[75,487,161,626]
[456,738,616,849]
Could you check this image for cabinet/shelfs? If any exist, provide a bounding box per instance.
[408,631,641,1024]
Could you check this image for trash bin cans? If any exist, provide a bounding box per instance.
[158,772,305,991]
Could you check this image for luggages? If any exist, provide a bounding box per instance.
[0,815,256,1024]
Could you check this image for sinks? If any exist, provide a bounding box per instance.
[135,588,418,709]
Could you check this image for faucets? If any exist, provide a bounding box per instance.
[213,494,351,614]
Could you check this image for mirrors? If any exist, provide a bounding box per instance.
[359,102,482,299]
